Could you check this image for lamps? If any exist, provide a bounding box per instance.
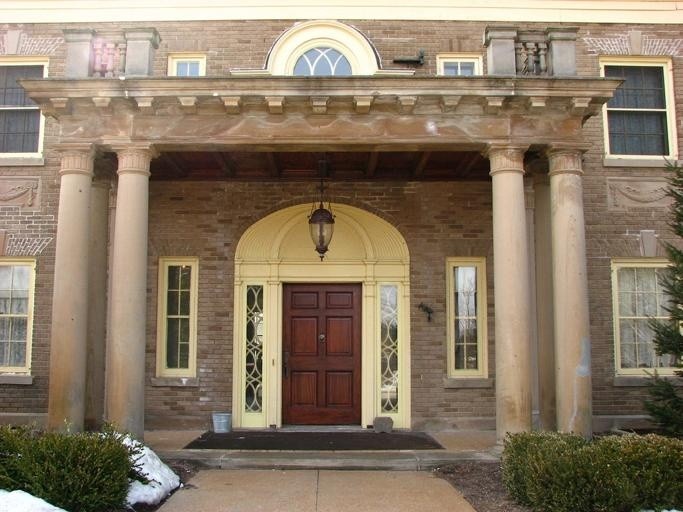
[306,172,335,263]
